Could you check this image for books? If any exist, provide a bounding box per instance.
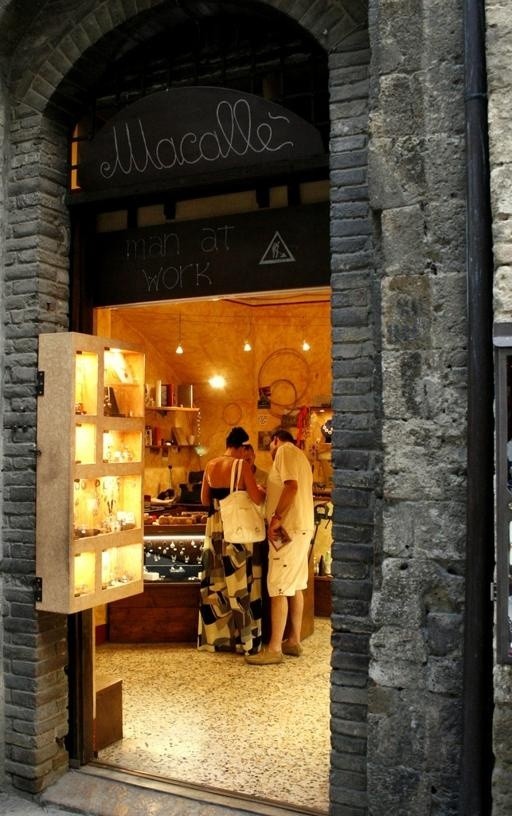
[270,527,293,551]
[144,378,194,445]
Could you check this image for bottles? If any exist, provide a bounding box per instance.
[167,383,174,407]
[152,427,162,447]
[184,383,194,408]
[144,424,153,448]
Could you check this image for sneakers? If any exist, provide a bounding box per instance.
[243,645,287,665]
[281,641,304,655]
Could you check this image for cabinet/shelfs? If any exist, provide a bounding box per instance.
[307,404,332,496]
[36,330,150,617]
[106,521,215,647]
[144,405,201,457]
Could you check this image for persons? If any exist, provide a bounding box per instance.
[245,430,317,666]
[244,443,269,485]
[197,425,265,654]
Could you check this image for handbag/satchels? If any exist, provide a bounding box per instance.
[218,457,268,545]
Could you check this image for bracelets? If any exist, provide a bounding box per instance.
[272,511,281,521]
